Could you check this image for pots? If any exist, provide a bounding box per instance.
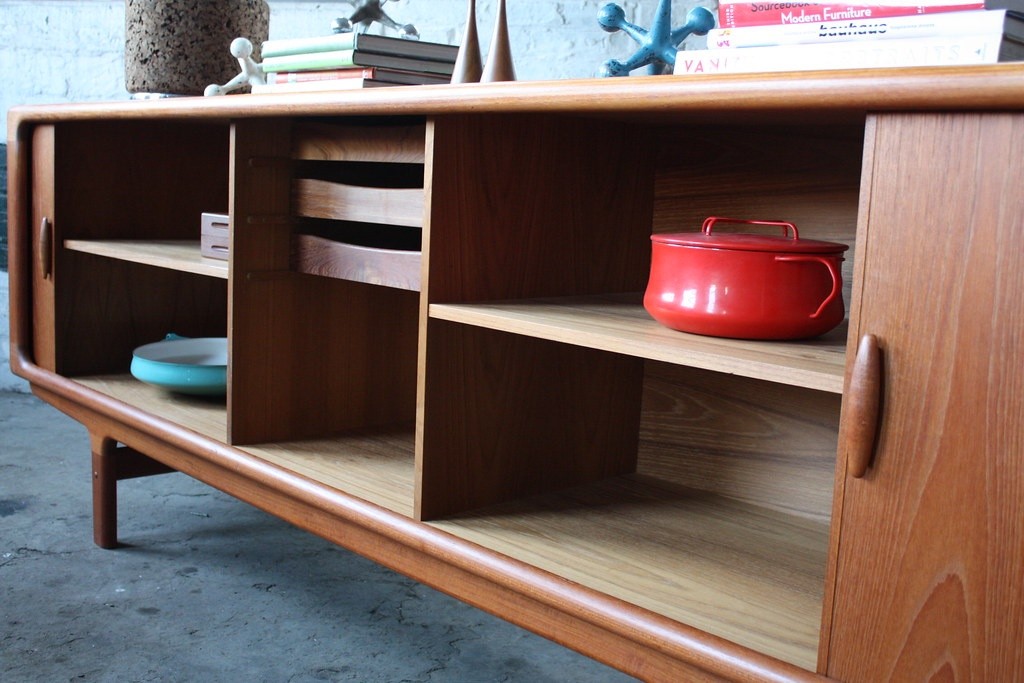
[644,216,849,342]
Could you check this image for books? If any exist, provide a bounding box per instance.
[672,0,1023,76]
[251,32,461,88]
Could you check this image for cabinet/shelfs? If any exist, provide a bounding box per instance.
[8,60,1024,683]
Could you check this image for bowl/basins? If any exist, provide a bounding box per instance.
[130,338,227,397]
